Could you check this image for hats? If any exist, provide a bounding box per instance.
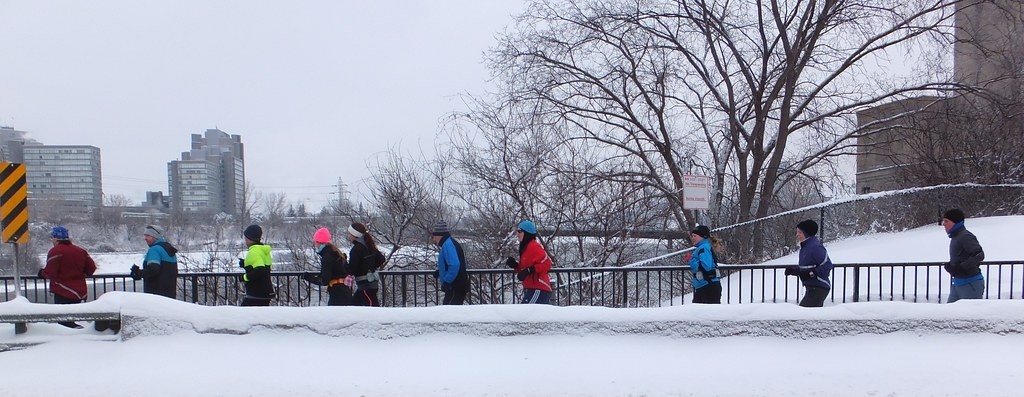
[313,227,331,243]
[50,226,69,240]
[795,220,818,237]
[432,219,450,236]
[144,225,164,239]
[944,209,965,223]
[517,221,536,234]
[244,225,262,241]
[692,225,710,239]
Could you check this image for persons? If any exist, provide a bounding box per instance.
[432,219,470,305]
[685,225,722,304]
[300,228,352,306]
[130,225,178,298]
[38,226,97,328]
[943,209,985,303]
[784,220,833,306]
[506,220,552,304]
[346,223,385,307]
[239,225,275,307]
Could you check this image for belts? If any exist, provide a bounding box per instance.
[356,276,368,282]
[692,274,714,279]
[326,278,343,287]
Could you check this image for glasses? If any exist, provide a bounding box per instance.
[146,225,160,234]
[517,230,524,233]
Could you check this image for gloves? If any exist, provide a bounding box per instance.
[506,256,519,269]
[800,267,817,281]
[433,269,440,279]
[37,268,44,279]
[304,272,311,281]
[131,264,141,280]
[785,266,797,274]
[441,282,450,292]
[517,266,534,281]
[685,254,692,261]
[238,258,244,268]
